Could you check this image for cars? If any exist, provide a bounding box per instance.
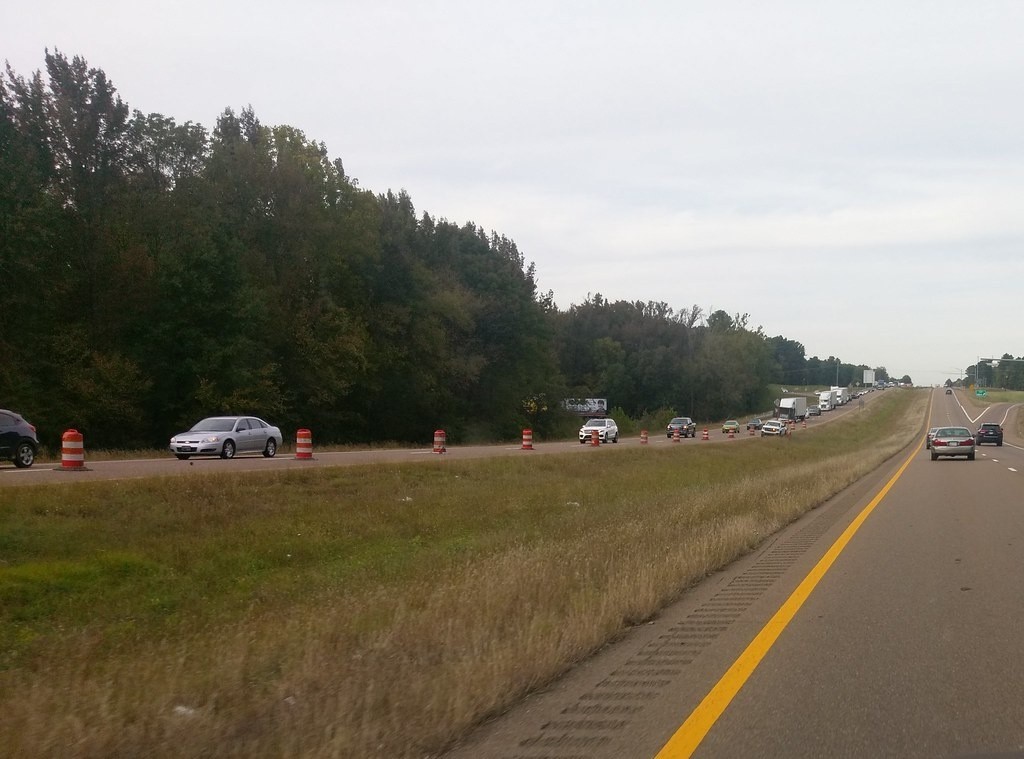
[169,415,283,460]
[925,426,976,461]
[850,379,913,399]
[0,408,41,468]
[761,420,788,437]
[666,417,697,438]
[806,405,822,420]
[746,418,764,429]
[721,420,740,433]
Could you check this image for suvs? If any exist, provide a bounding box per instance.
[578,418,619,444]
[977,421,1005,450]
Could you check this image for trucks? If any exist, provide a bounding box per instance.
[819,385,852,412]
[779,396,807,423]
